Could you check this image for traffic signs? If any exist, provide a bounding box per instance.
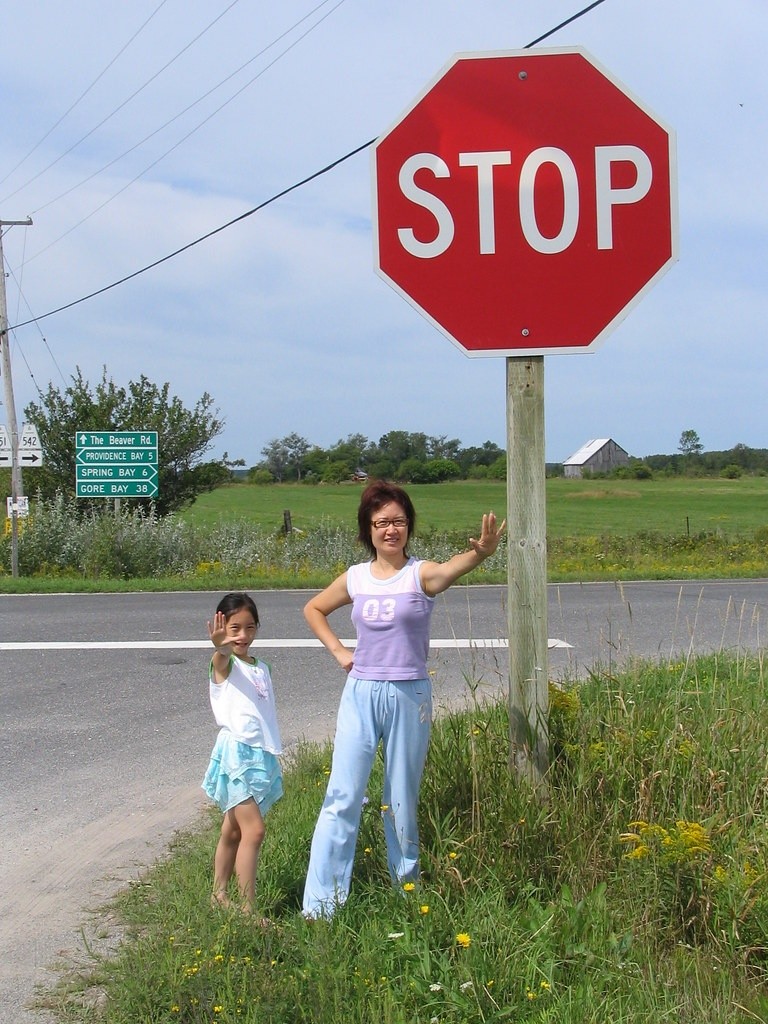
[0,424,14,470]
[74,462,161,483]
[75,481,161,500]
[17,422,45,469]
[75,450,161,463]
[72,430,161,449]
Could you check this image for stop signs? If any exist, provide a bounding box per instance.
[374,48,675,361]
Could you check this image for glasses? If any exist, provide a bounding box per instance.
[369,518,409,528]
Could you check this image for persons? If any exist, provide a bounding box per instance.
[302,477,506,921]
[208,591,284,932]
